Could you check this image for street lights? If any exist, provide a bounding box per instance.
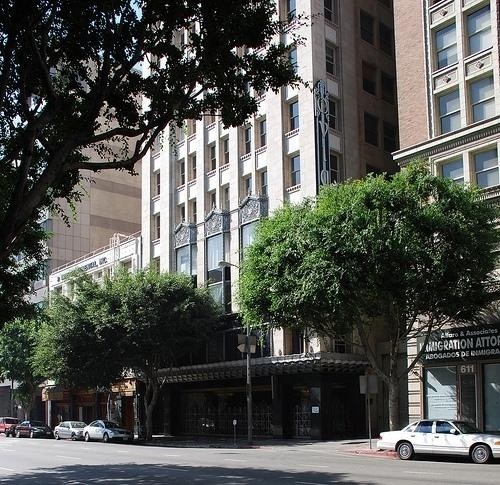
[216,260,253,445]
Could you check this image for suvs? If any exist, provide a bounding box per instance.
[0,417,21,437]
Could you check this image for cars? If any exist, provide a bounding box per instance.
[378,419,500,463]
[83,419,132,443]
[14,420,53,439]
[53,420,87,441]
